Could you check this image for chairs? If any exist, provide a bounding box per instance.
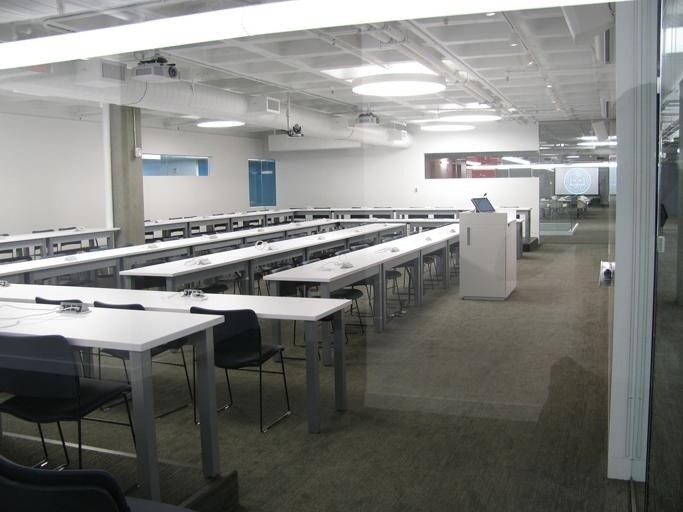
[539,195,591,221]
[0,455,131,512]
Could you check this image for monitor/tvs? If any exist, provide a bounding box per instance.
[471,198,496,213]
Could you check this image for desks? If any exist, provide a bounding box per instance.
[0,205,533,502]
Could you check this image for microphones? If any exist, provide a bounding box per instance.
[473,193,487,212]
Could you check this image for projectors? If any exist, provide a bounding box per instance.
[356,115,380,125]
[131,65,182,84]
